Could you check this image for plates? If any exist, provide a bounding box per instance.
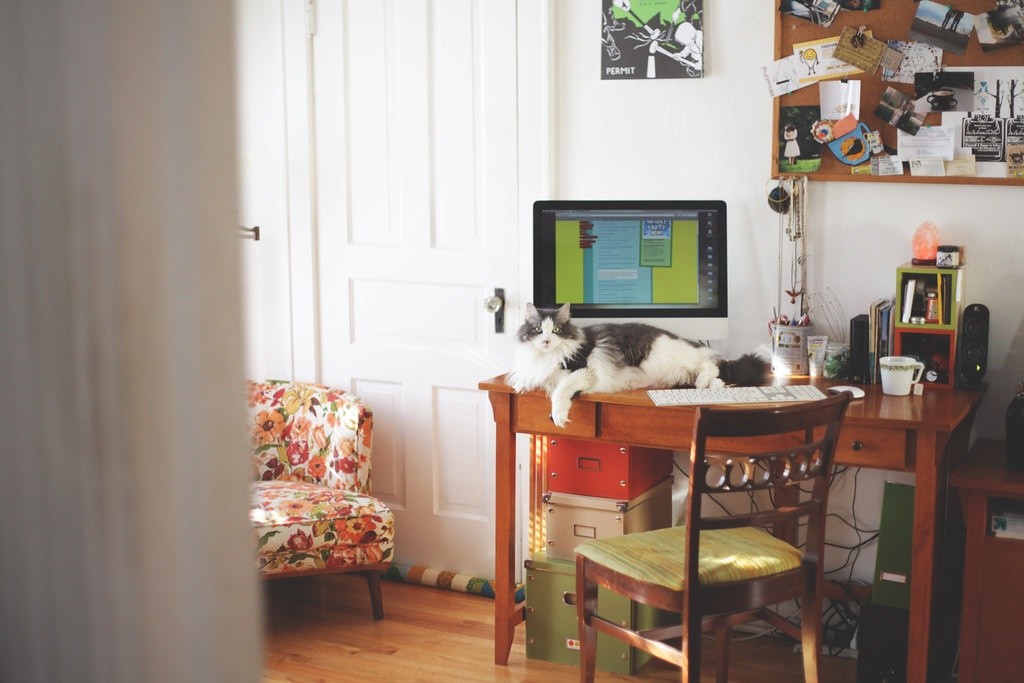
[931,99,958,109]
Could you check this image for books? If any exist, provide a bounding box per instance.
[867,296,894,387]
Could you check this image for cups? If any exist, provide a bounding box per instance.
[927,89,955,104]
[879,356,924,396]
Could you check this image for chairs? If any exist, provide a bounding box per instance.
[247,379,393,620]
[576,391,853,682]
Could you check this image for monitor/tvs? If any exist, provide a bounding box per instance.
[532,200,728,340]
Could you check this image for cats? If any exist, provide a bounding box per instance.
[505,303,791,429]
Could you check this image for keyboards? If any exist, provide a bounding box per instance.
[647,384,828,406]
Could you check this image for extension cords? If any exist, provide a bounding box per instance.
[793,643,859,660]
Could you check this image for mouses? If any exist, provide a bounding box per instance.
[828,385,865,398]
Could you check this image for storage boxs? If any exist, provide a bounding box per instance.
[544,478,681,561]
[523,557,668,674]
[543,434,675,501]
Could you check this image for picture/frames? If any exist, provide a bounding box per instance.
[771,0,1022,187]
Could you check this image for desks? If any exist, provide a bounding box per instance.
[944,438,1023,683]
[479,366,991,683]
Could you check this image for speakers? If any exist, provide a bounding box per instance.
[861,482,951,683]
[956,304,990,389]
